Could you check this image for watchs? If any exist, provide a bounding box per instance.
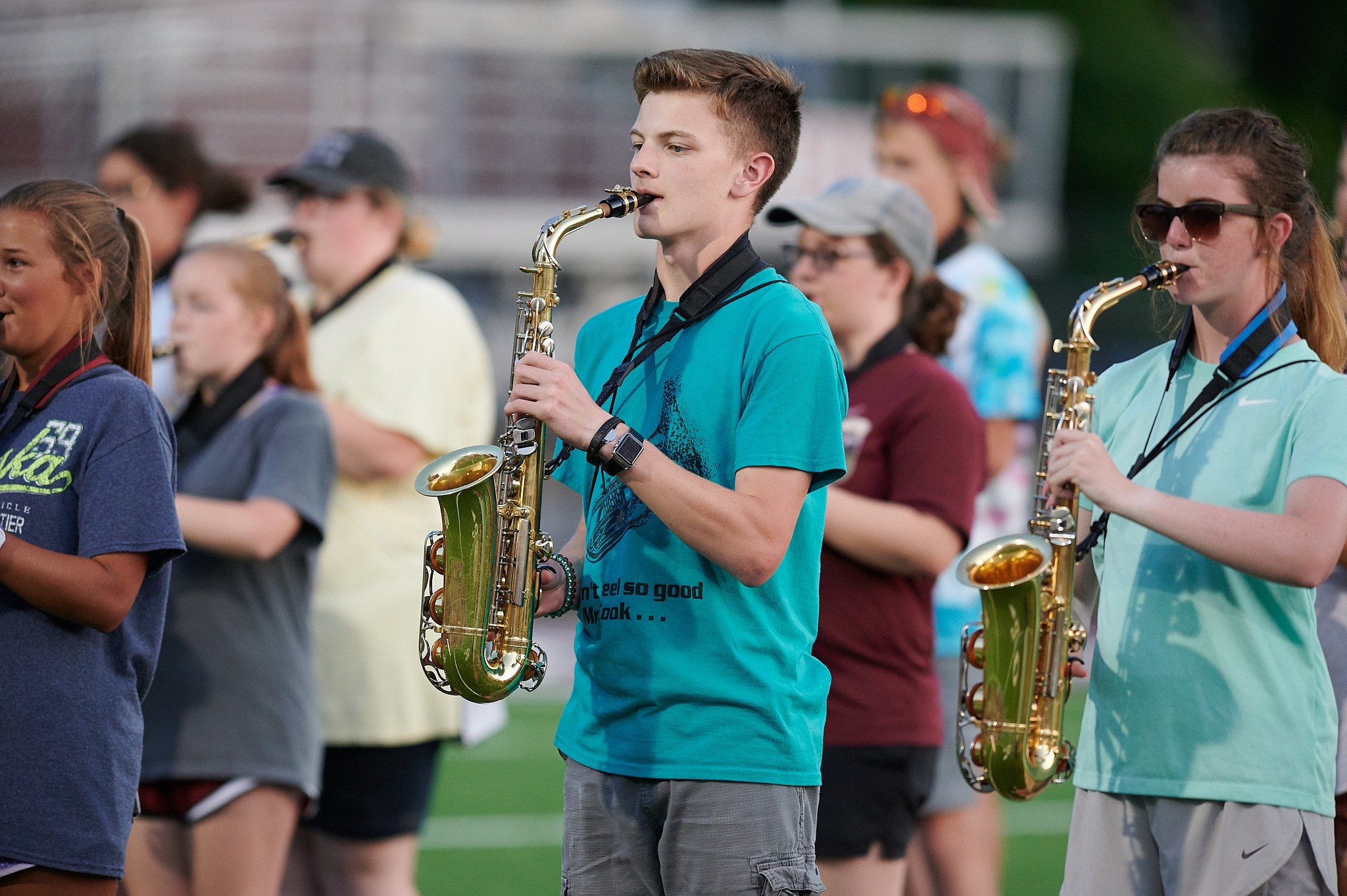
[600,428,646,476]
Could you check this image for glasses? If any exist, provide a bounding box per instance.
[1128,199,1284,242]
[777,241,881,274]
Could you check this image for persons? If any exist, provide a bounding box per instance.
[86,121,332,896]
[865,83,1056,895]
[1057,108,1347,896]
[774,175,983,896]
[465,47,849,896]
[0,182,172,896]
[1314,142,1347,896]
[271,130,497,896]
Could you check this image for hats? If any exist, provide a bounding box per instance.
[872,79,1004,233]
[264,128,419,199]
[765,172,938,289]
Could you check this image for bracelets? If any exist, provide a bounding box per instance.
[586,416,625,468]
[545,553,577,619]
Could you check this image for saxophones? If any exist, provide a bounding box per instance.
[408,188,649,709]
[136,347,174,366]
[233,234,306,255]
[950,255,1191,807]
